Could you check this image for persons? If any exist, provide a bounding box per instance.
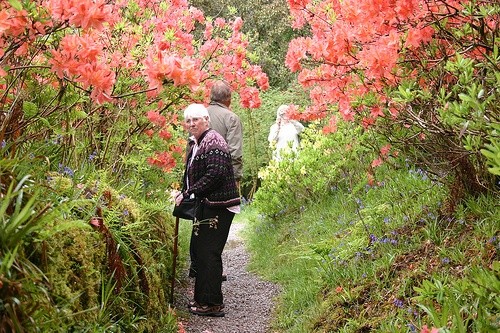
[268,105,305,162]
[187,79,244,281]
[172,104,241,317]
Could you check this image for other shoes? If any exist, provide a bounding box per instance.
[190,264,227,281]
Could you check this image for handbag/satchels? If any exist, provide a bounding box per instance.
[172,195,200,220]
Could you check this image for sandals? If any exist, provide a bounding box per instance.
[190,304,225,317]
[188,301,200,308]
